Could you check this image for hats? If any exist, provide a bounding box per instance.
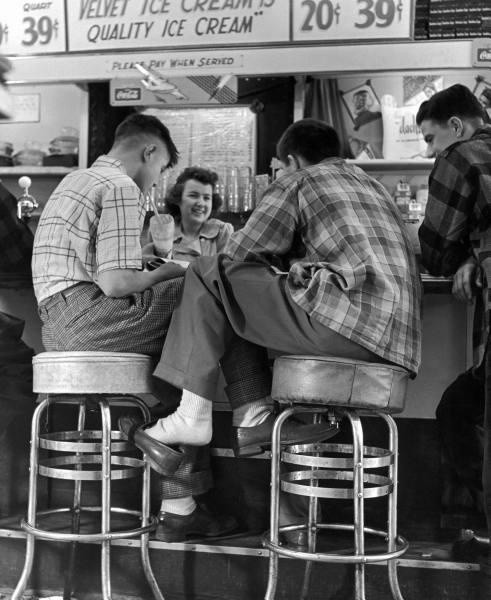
[417,82,486,124]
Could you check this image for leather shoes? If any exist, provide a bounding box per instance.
[119,414,186,478]
[156,504,240,544]
[228,411,340,460]
[282,527,310,553]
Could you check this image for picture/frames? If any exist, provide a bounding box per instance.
[340,79,383,131]
[470,76,491,122]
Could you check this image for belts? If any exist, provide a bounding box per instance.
[39,281,94,315]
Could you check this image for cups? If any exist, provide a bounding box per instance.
[210,165,270,212]
[149,214,173,257]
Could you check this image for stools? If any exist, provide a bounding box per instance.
[10,352,166,600]
[263,354,412,600]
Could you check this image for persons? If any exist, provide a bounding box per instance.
[32,113,423,545]
[353,90,381,127]
[478,87,491,109]
[417,82,491,574]
[423,83,437,100]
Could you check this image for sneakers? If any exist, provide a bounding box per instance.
[432,526,491,554]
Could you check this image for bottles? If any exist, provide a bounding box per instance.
[416,183,428,216]
